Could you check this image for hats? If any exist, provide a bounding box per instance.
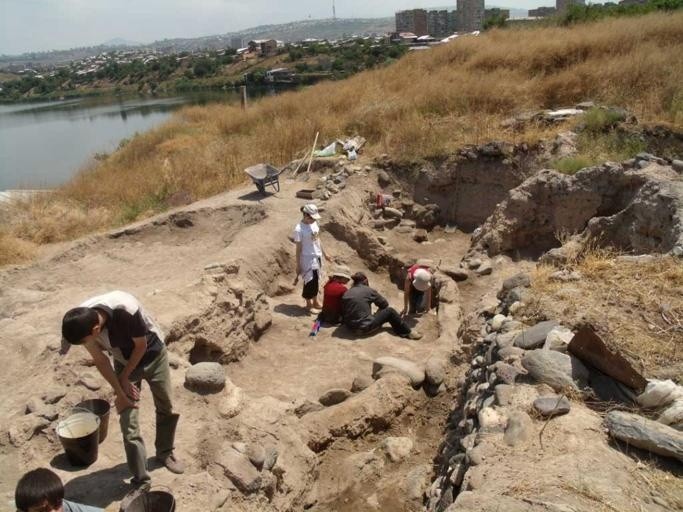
[330,265,352,280]
[411,268,432,292]
[302,203,322,221]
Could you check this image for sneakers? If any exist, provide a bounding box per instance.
[401,330,424,340]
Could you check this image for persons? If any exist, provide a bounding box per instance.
[294,204,332,314]
[14,467,115,511]
[341,271,424,339]
[316,265,350,325]
[399,263,431,319]
[61,290,185,511]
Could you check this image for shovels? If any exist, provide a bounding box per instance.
[286,150,310,185]
[302,131,320,181]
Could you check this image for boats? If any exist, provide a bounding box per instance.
[263,68,297,85]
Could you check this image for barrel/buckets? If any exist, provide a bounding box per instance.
[55,408,102,464]
[71,399,110,443]
[119,484,176,512]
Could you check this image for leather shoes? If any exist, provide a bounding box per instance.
[155,450,184,473]
[120,482,150,511]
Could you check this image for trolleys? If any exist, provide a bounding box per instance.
[242,161,289,197]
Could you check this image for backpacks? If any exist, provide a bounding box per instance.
[415,257,433,268]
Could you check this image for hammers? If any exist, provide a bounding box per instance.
[292,273,302,285]
